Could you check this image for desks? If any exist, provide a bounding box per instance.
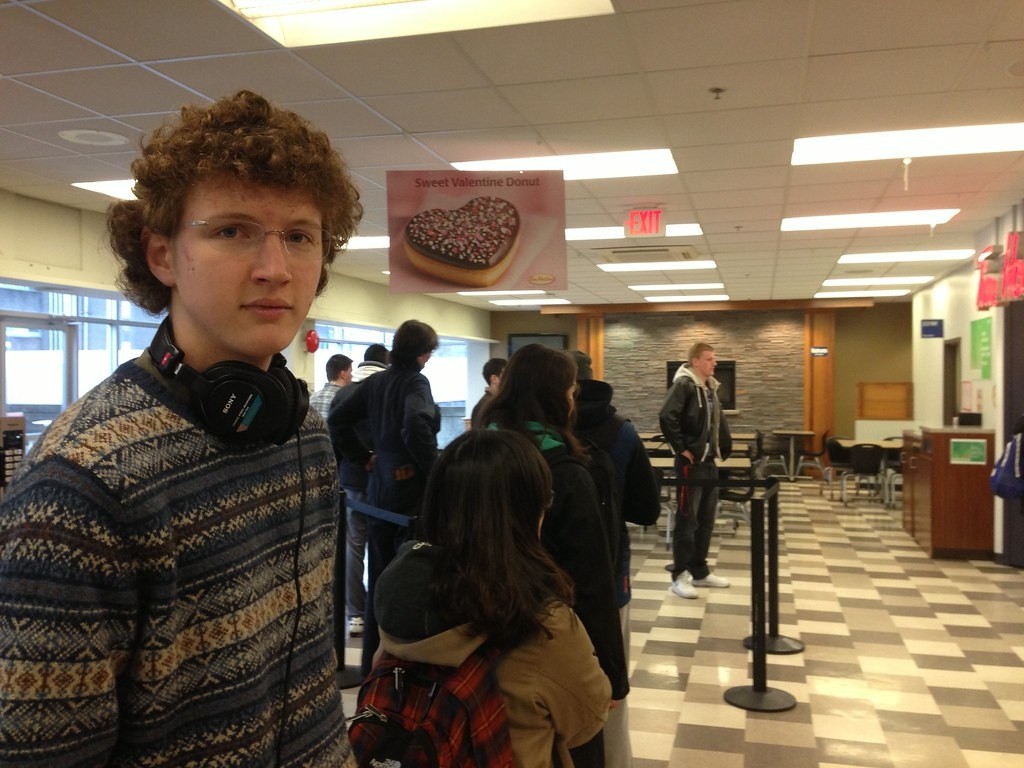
[648,458,751,523]
[837,439,903,450]
[773,429,816,480]
[643,441,748,457]
[636,432,756,440]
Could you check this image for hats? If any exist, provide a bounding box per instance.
[571,350,593,381]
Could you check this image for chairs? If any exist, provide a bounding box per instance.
[721,430,903,534]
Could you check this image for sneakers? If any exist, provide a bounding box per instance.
[672,570,699,599]
[692,574,731,588]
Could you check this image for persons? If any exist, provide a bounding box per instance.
[478,344,631,768]
[658,343,729,599]
[373,428,617,768]
[326,320,442,681]
[569,348,663,768]
[470,357,508,428]
[330,343,389,637]
[309,354,354,420]
[0,90,361,768]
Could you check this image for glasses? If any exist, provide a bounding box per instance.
[174,218,336,263]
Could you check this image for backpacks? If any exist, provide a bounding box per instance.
[573,413,631,576]
[990,433,1024,500]
[346,593,562,768]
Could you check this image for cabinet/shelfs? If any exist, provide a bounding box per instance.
[901,426,997,562]
